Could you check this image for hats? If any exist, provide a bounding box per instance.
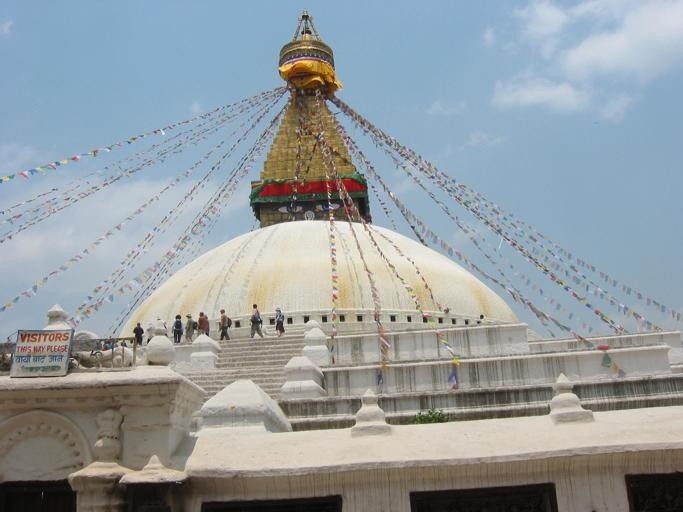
[186,313,191,317]
[275,308,281,311]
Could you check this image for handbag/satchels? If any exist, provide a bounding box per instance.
[194,322,197,329]
[228,318,232,327]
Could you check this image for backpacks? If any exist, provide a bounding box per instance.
[176,319,181,328]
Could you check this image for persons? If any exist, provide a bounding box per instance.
[94,338,128,351]
[248,302,264,338]
[272,307,284,337]
[217,308,230,341]
[170,314,183,344]
[196,311,208,337]
[183,312,194,343]
[132,322,144,346]
[203,314,208,337]
